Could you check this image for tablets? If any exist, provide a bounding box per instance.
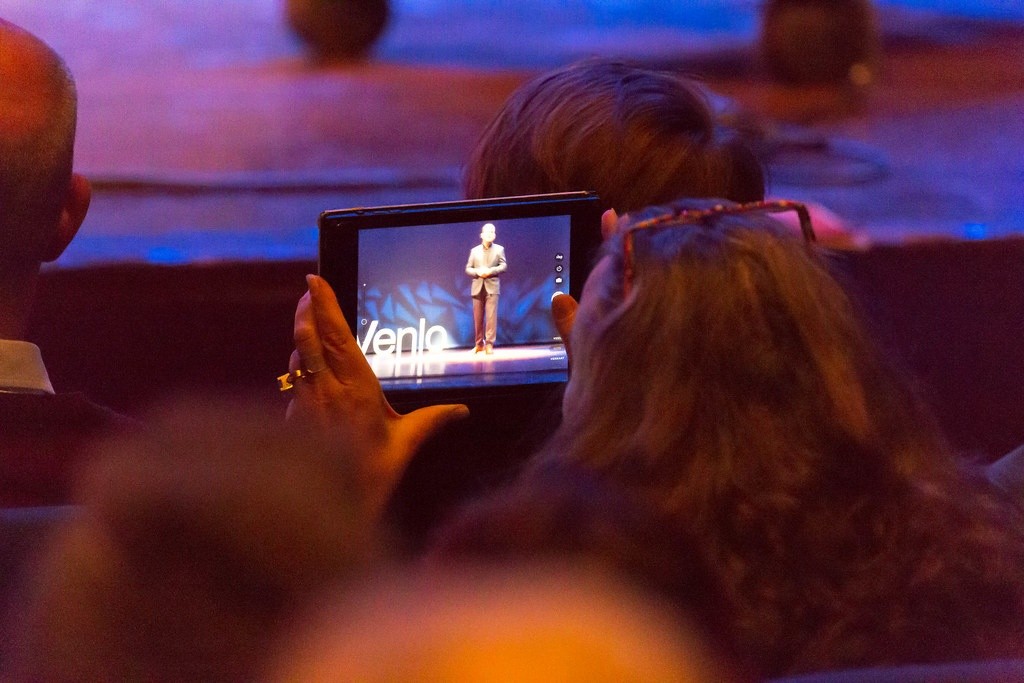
[320,193,606,403]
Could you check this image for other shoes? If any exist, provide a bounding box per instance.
[486,344,493,354]
[467,345,484,354]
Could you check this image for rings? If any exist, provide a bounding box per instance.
[283,367,328,383]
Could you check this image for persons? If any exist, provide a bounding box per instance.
[1,18,146,511]
[465,222,507,355]
[27,58,1024,683]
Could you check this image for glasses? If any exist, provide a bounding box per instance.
[622,199,819,281]
